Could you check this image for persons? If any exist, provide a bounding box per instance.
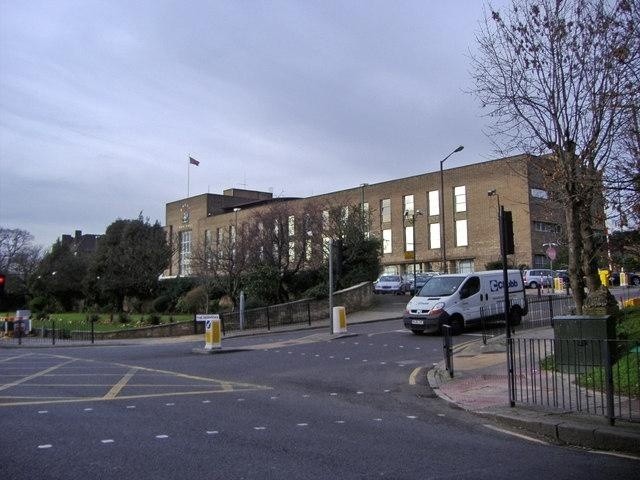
[0,318,10,335]
[7,318,14,337]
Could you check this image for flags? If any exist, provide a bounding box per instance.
[190,157,200,166]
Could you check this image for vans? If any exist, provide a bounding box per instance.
[524,267,556,288]
[402,269,529,338]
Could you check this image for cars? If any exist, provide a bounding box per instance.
[556,269,569,288]
[373,272,405,296]
[405,270,439,294]
[609,271,640,287]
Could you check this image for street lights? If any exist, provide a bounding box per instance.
[543,242,559,275]
[440,143,465,270]
[403,208,424,294]
[486,188,502,255]
